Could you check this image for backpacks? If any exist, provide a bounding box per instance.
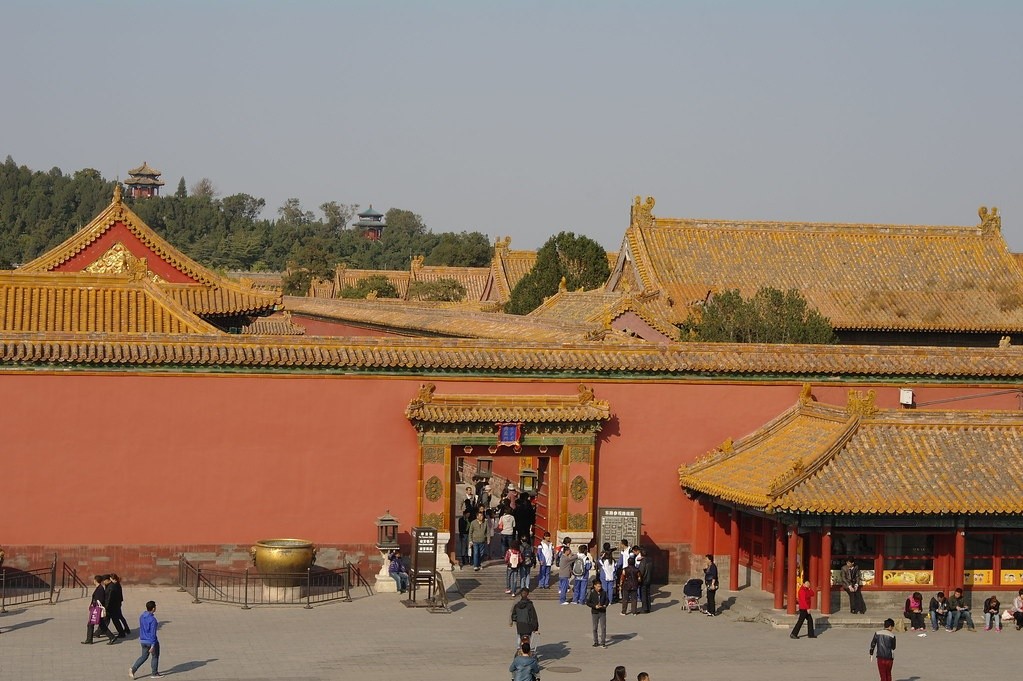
[521,543,534,568]
[572,554,587,576]
[508,549,520,569]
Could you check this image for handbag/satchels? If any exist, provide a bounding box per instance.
[706,576,719,591]
[89,600,106,625]
[556,545,565,567]
[497,516,503,530]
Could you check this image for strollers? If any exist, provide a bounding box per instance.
[681,578,703,613]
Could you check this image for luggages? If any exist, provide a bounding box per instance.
[528,631,540,652]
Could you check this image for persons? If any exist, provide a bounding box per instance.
[80,574,131,645]
[128,601,164,679]
[946,588,977,633]
[1011,589,1023,630]
[610,666,627,681]
[790,579,817,639]
[700,554,718,617]
[388,550,410,594]
[840,556,865,614]
[458,476,654,627]
[509,643,539,681]
[637,672,650,681]
[982,596,1001,633]
[511,587,539,650]
[869,618,896,681]
[902,591,928,632]
[586,579,610,649]
[929,592,953,633]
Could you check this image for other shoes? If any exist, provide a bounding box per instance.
[538,586,550,591]
[107,637,117,645]
[910,627,1023,632]
[703,610,715,618]
[81,641,93,644]
[638,610,650,614]
[129,667,136,680]
[93,633,100,638]
[459,555,506,572]
[790,633,818,639]
[619,610,637,616]
[558,588,571,594]
[123,630,130,634]
[116,634,126,638]
[504,589,515,597]
[398,589,409,595]
[851,609,865,614]
[592,643,606,647]
[150,674,165,679]
[560,597,632,605]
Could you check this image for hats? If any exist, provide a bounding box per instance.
[485,485,491,490]
[507,483,515,490]
